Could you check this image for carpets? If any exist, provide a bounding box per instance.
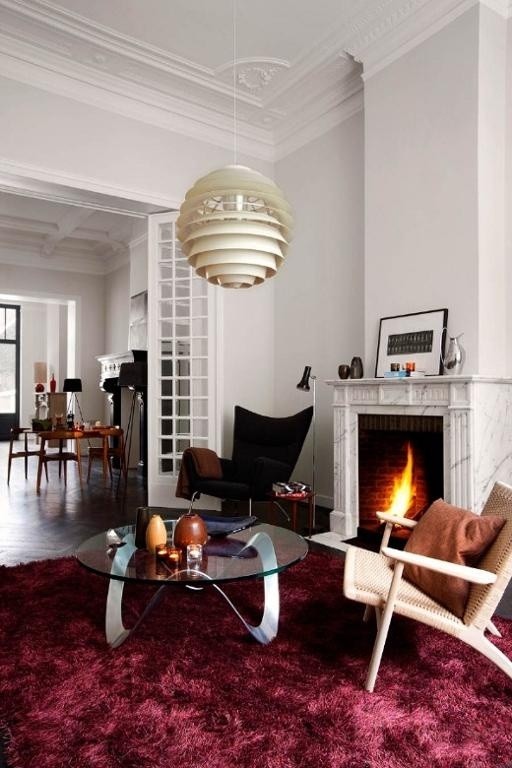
[3,545,512,768]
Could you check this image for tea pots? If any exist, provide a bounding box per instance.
[441,326,465,374]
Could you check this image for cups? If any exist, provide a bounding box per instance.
[156,544,169,559]
[168,548,182,566]
[187,544,203,563]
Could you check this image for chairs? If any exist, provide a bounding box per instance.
[342,480,512,693]
[87,428,125,489]
[37,432,87,494]
[8,427,50,487]
[183,405,312,534]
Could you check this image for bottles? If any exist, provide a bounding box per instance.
[135,506,148,549]
[50,373,56,393]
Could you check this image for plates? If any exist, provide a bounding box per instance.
[196,514,258,539]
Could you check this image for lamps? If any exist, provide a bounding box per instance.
[61,378,92,454]
[33,360,47,392]
[297,366,323,529]
[177,2,295,290]
[117,361,148,492]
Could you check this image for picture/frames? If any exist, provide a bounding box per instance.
[375,309,448,377]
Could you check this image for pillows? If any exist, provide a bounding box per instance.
[391,499,506,616]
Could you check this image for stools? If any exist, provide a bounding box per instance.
[269,489,314,536]
